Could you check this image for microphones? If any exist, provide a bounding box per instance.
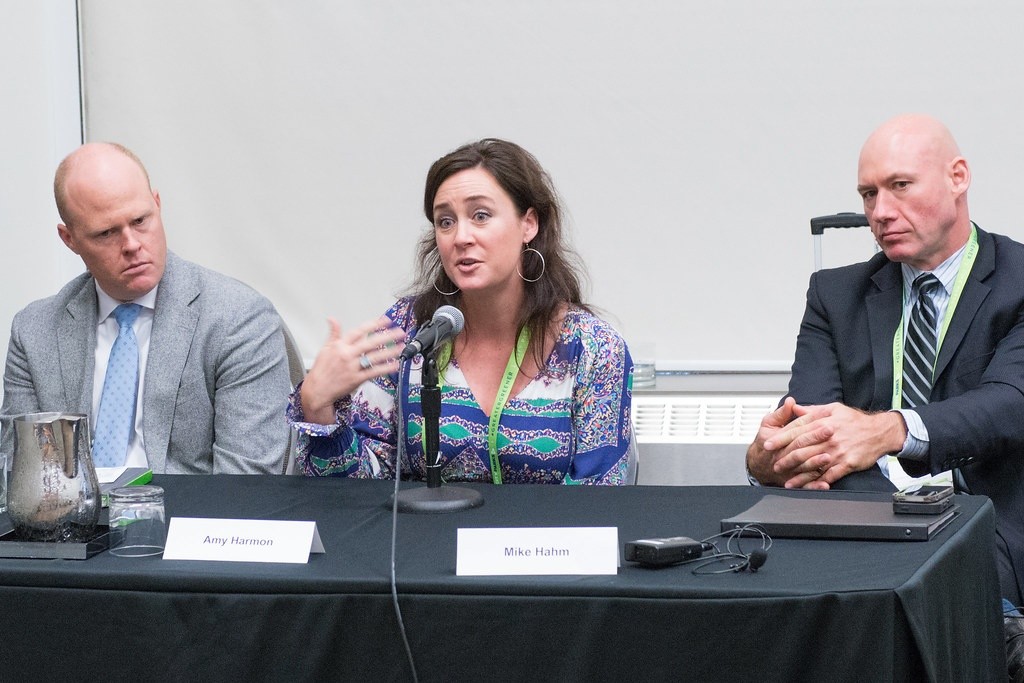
[400,305,464,359]
[749,549,767,573]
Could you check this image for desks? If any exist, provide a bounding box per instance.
[0,474,1003,683]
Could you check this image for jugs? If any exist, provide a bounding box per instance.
[8,412,102,540]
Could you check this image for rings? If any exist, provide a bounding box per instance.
[360,353,371,368]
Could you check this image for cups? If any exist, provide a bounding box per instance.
[0,453,8,513]
[107,486,167,557]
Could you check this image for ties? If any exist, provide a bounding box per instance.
[92,304,143,466]
[901,274,941,409]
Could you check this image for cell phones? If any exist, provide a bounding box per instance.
[892,484,955,514]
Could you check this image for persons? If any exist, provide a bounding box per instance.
[3,139,293,474]
[283,140,636,485]
[745,114,1024,617]
